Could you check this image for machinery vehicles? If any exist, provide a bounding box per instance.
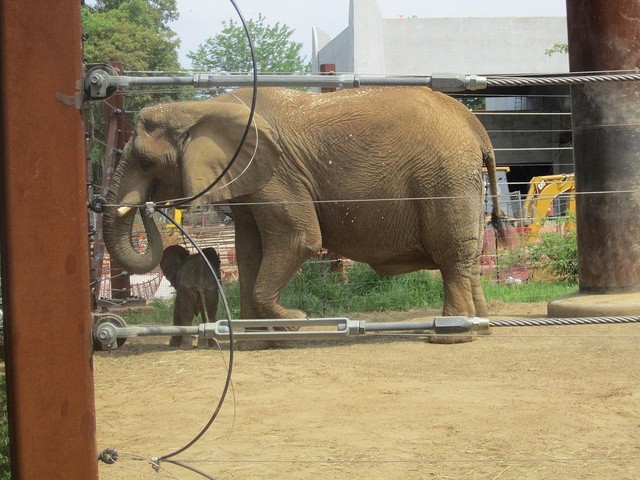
[482,167,575,281]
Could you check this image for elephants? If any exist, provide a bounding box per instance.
[160,244,221,351]
[102,82,510,352]
